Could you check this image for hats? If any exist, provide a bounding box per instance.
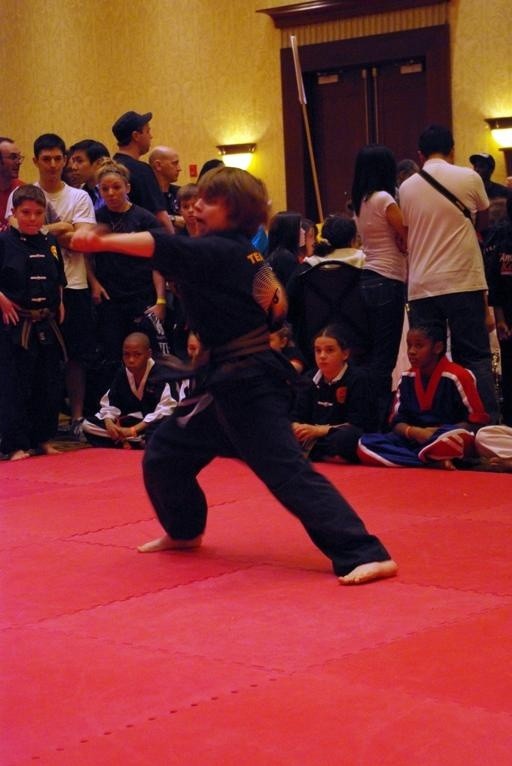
[469,153,496,171]
[111,111,153,144]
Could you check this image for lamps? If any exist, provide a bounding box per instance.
[215,142,257,171]
[483,116,512,152]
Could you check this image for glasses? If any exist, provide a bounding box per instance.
[1,154,25,162]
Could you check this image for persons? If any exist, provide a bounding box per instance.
[69,166,398,586]
[0,111,512,470]
